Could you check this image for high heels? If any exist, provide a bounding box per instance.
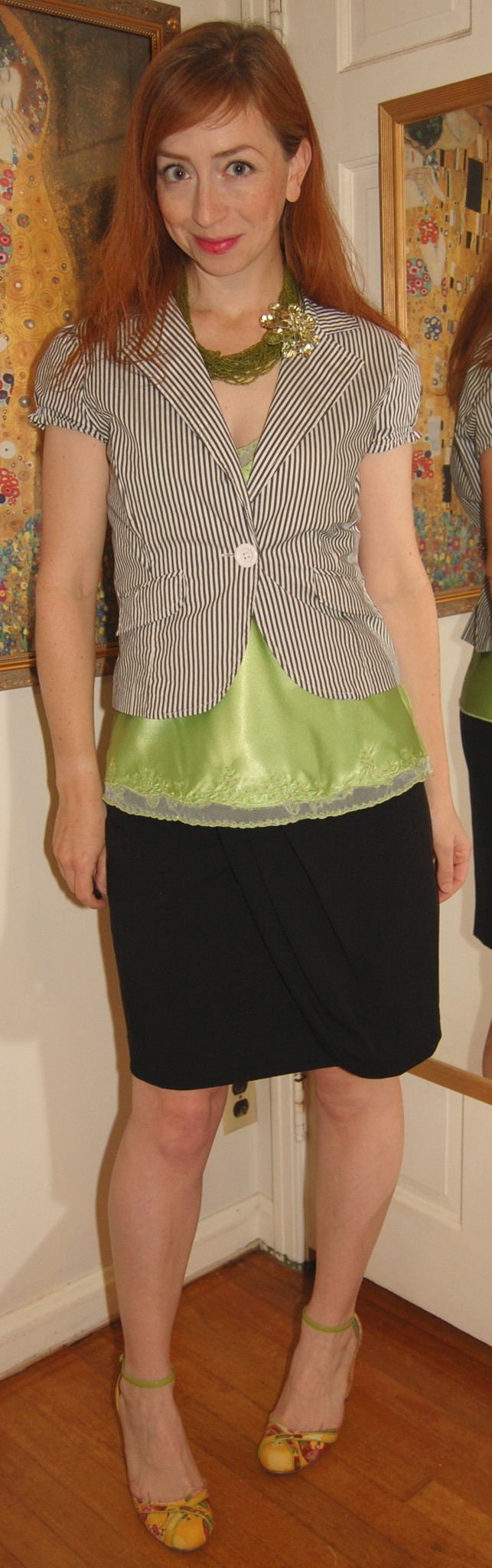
[257,1305,363,1477]
[115,1353,214,1554]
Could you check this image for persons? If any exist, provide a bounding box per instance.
[446,252,492,1080]
[31,22,472,1551]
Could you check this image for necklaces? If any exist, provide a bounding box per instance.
[170,257,318,385]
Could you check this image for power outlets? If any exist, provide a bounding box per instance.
[218,1081,258,1135]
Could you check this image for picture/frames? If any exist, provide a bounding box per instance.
[0,0,181,693]
[379,72,492,617]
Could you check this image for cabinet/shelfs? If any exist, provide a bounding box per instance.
[285,613,492,1347]
[0,676,285,1384]
